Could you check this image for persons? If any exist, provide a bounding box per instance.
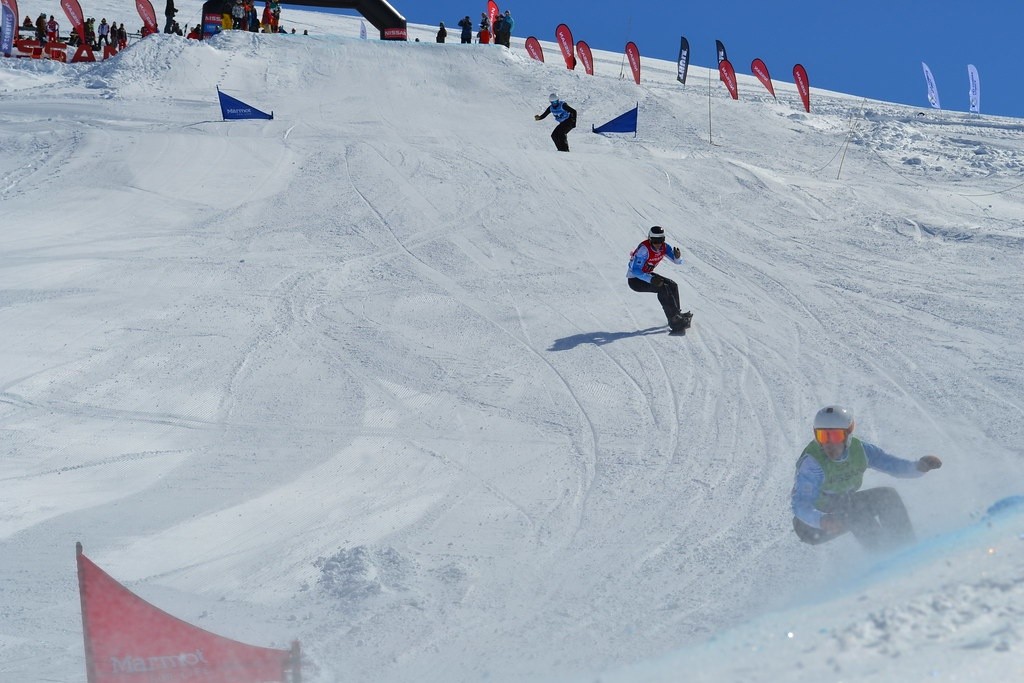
[791,405,942,561]
[627,226,690,325]
[415,10,513,50]
[535,93,577,152]
[25,0,308,52]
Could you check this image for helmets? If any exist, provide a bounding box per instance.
[813,405,854,448]
[648,225,665,253]
[549,93,560,108]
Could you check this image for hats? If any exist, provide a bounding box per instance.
[504,9,511,15]
[465,15,469,19]
[495,14,501,19]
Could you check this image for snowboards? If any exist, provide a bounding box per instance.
[673,313,694,331]
[834,495,1024,580]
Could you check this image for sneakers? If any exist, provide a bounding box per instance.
[668,311,693,330]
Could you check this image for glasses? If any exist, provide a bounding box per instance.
[551,100,559,104]
[650,239,663,244]
[813,428,847,445]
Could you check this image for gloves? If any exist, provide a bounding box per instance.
[914,455,941,474]
[673,247,681,260]
[651,274,665,287]
